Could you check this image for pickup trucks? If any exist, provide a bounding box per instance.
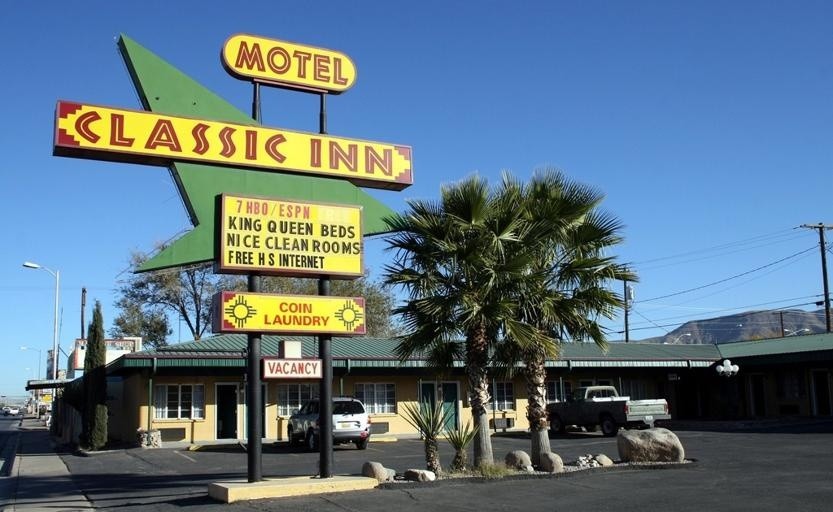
[544,383,671,438]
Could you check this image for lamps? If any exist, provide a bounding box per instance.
[715,359,739,378]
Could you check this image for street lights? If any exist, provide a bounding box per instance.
[671,333,692,344]
[714,359,741,422]
[784,327,810,337]
[19,346,42,419]
[783,328,798,336]
[19,260,61,408]
[25,365,35,416]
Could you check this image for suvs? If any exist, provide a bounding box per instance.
[285,395,370,451]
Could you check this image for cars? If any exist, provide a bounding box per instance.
[0,405,25,417]
